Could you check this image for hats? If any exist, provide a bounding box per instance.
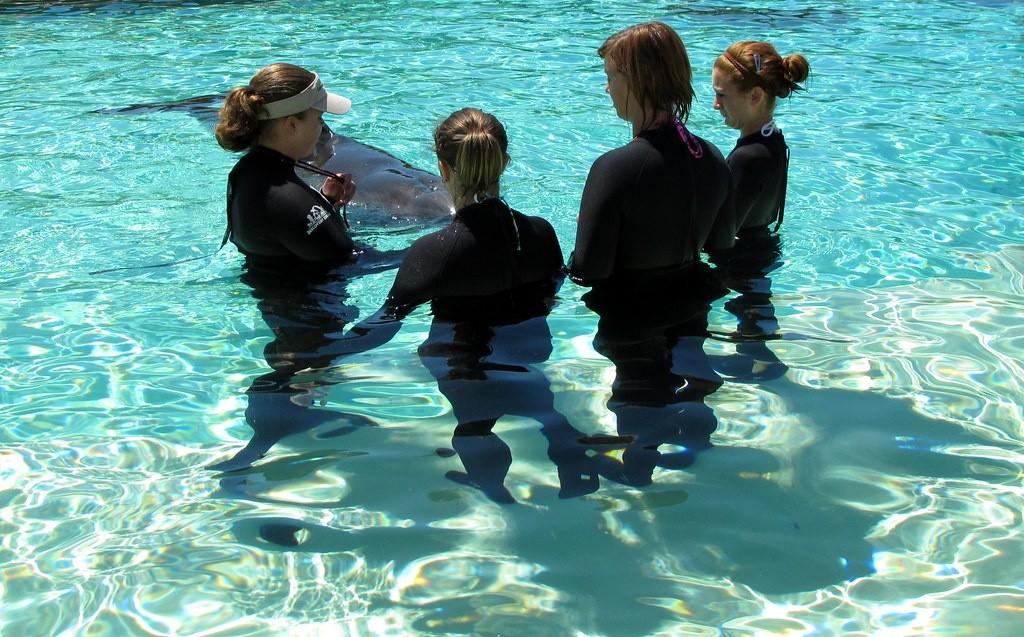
[260,72,352,119]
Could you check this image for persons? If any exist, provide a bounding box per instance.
[709,39,811,233]
[389,105,567,304]
[213,62,355,262]
[563,20,737,280]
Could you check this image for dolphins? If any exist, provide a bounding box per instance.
[93,93,463,223]
